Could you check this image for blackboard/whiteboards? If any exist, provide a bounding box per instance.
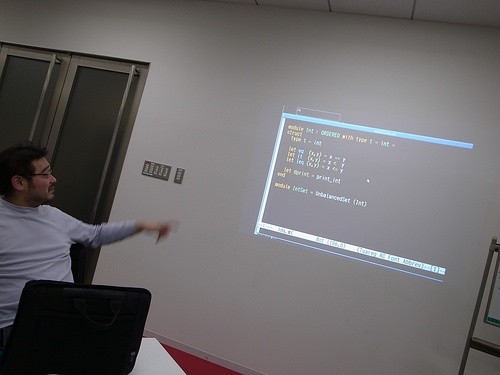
[468,239,499,358]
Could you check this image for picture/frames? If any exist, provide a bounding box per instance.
[483,252,500,328]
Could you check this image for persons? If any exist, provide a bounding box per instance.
[1,141,180,348]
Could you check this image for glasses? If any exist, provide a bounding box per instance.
[20,170,52,178]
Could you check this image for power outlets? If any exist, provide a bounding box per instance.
[142,160,185,185]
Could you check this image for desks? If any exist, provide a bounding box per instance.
[125,337,188,375]
